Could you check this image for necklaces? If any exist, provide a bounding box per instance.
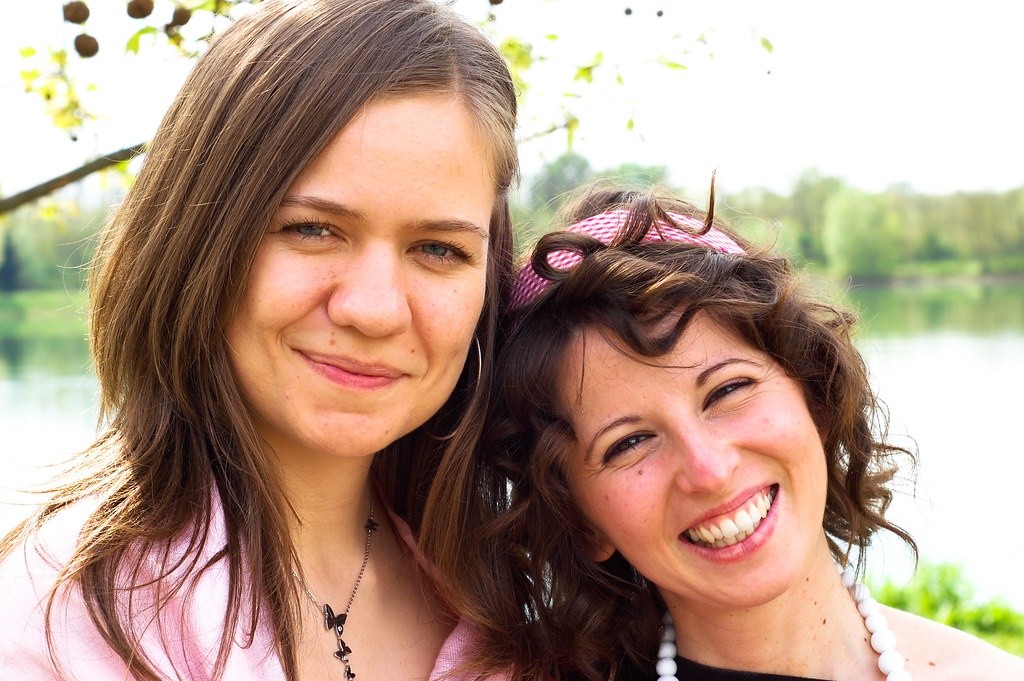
[291,488,377,681]
[656,569,906,681]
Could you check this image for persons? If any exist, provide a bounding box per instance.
[0,0,546,681]
[494,185,1024,681]
